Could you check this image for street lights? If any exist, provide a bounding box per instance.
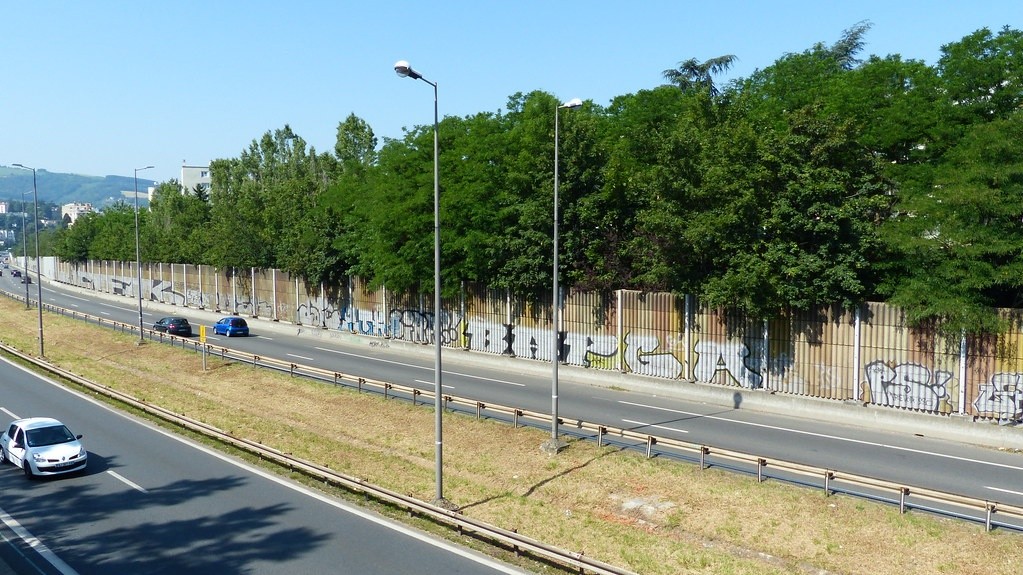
[134,165,156,344]
[551,96,584,435]
[11,162,45,358]
[392,59,445,503]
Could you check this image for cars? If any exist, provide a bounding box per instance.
[21,275,31,284]
[0,254,22,278]
[153,316,192,338]
[0,416,88,480]
[213,316,250,337]
